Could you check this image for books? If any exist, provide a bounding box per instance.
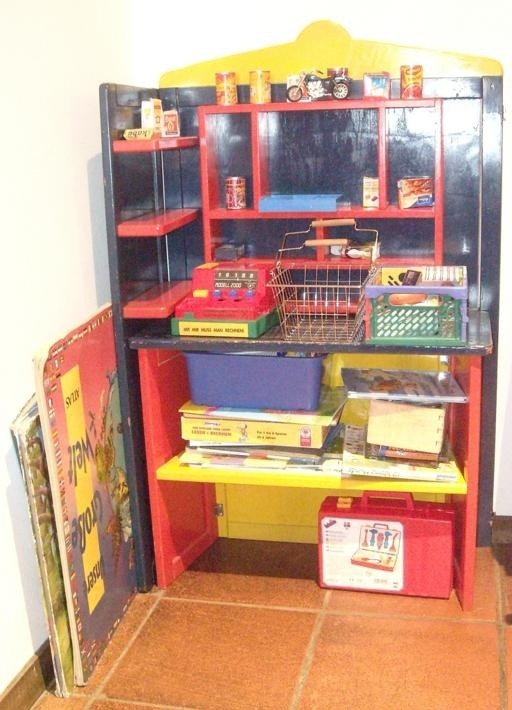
[38,298,139,683]
[338,361,468,406]
[177,386,348,469]
[8,386,78,702]
[342,422,457,481]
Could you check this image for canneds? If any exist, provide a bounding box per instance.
[400,65,422,100]
[249,69,272,103]
[215,72,239,105]
[224,175,248,210]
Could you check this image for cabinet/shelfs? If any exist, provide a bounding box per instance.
[98,66,505,614]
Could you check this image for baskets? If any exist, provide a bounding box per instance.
[265,218,378,342]
[365,265,470,345]
